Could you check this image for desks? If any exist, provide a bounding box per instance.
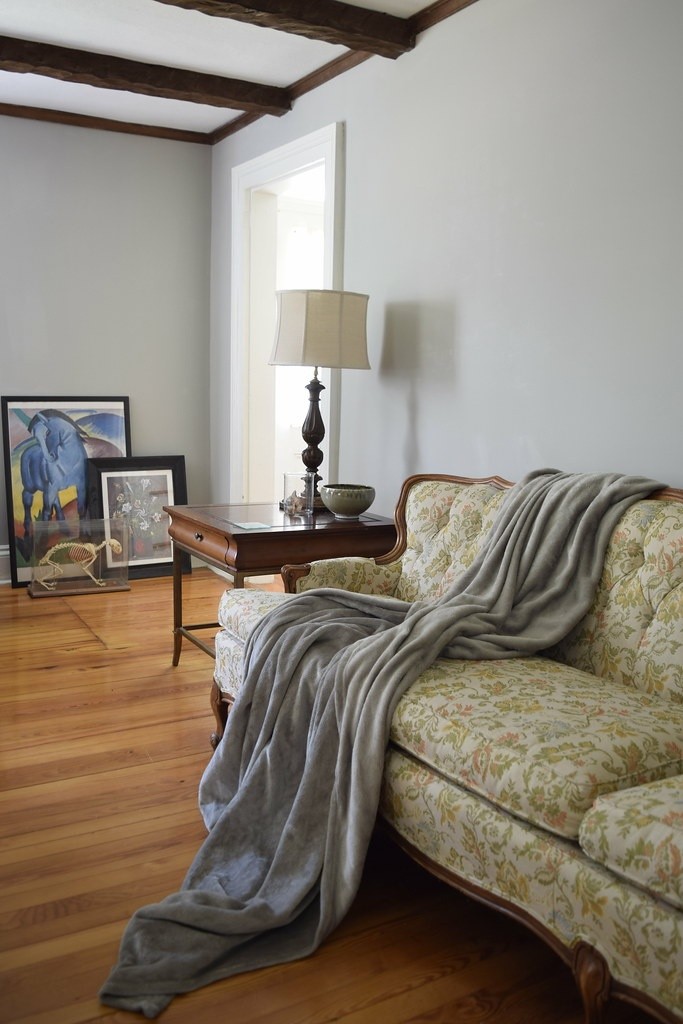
[163,500,397,666]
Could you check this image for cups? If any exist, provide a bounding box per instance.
[284,473,314,516]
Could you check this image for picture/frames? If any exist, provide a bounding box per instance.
[1,396,132,588]
[83,454,193,582]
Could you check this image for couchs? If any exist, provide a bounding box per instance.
[209,474,683,1024]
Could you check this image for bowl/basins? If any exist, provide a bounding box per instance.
[321,484,376,518]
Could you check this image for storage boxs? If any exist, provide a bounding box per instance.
[27,517,132,600]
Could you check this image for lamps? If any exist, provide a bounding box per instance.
[265,288,373,512]
